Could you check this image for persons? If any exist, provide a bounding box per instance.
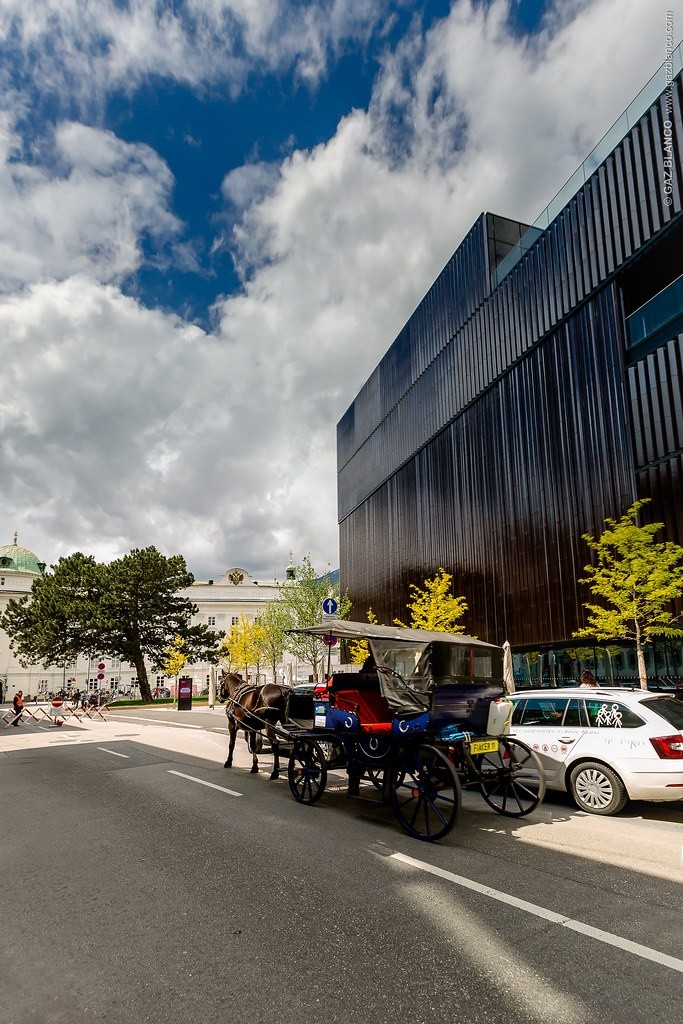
[359,641,394,673]
[580,670,600,687]
[13,687,117,726]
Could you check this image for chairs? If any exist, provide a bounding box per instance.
[524,702,547,727]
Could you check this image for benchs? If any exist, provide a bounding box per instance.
[313,673,393,735]
[394,684,507,743]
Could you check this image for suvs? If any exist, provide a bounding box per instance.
[150,687,170,697]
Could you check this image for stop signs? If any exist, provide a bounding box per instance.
[51,697,64,708]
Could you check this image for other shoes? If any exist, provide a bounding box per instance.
[12,723,20,726]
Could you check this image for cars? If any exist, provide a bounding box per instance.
[482,685,683,816]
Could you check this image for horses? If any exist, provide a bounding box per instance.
[218,669,296,780]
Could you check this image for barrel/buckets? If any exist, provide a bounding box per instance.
[487,696,513,736]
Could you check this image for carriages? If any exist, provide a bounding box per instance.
[216,619,547,842]
